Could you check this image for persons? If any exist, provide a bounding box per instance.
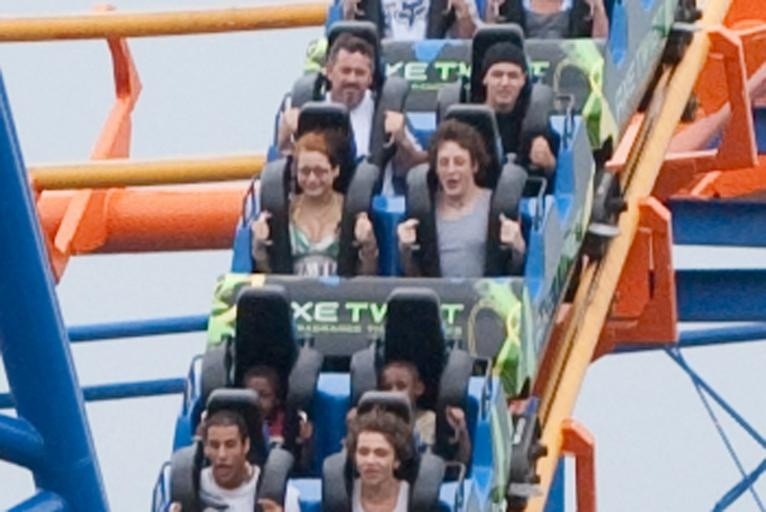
[481,39,558,196]
[167,410,301,512]
[343,0,477,40]
[278,33,419,197]
[342,405,420,511]
[397,120,527,276]
[381,359,471,463]
[487,0,608,39]
[242,365,312,449]
[253,131,379,276]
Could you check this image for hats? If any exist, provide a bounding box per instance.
[481,40,529,76]
